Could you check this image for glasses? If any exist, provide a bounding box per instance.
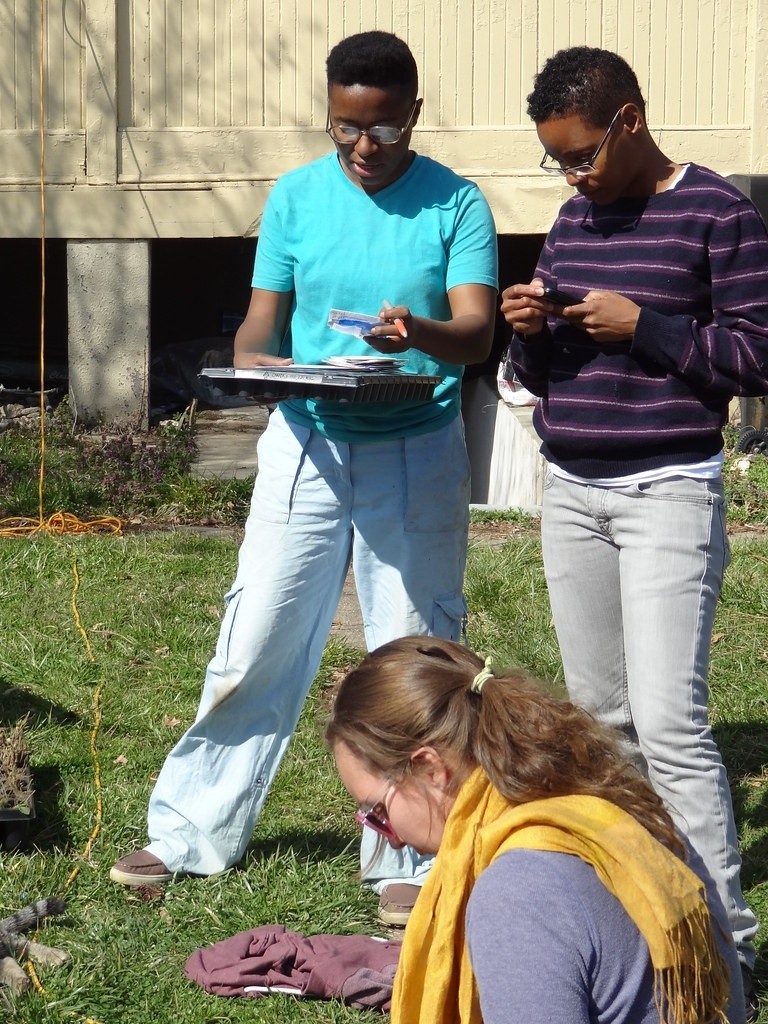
[354,762,407,841]
[540,107,621,176]
[326,100,417,145]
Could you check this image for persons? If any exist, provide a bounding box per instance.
[328,636,748,1023]
[503,46,768,1022]
[107,30,499,927]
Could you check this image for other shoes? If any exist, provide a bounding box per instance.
[377,883,422,924]
[741,964,757,1023]
[110,849,173,885]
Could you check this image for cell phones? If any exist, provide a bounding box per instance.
[541,288,586,306]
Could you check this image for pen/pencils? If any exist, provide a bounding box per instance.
[381,299,408,338]
